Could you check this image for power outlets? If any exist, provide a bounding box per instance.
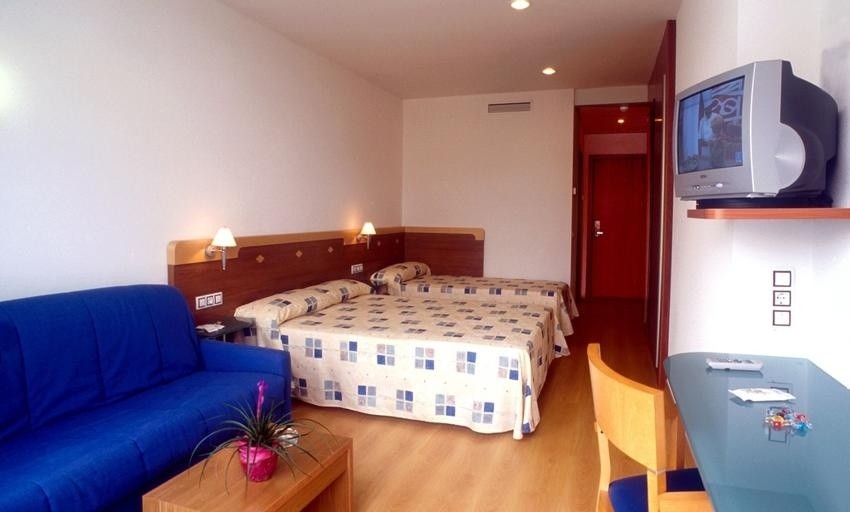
[774,291,791,307]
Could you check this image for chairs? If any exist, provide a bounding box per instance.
[587,343,713,512]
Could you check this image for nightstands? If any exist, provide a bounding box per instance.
[194,320,250,343]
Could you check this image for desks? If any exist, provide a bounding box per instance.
[664,351,850,512]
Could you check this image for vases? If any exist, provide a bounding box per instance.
[237,440,278,481]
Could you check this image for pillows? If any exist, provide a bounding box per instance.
[234,260,430,335]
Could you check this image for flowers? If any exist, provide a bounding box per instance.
[189,379,338,500]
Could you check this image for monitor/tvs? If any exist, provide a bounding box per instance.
[672,59,839,208]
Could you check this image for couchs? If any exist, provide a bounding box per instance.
[0,285,291,512]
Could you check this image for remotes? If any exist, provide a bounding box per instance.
[705,357,764,371]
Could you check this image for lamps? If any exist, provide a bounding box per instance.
[356,222,376,250]
[206,228,237,270]
